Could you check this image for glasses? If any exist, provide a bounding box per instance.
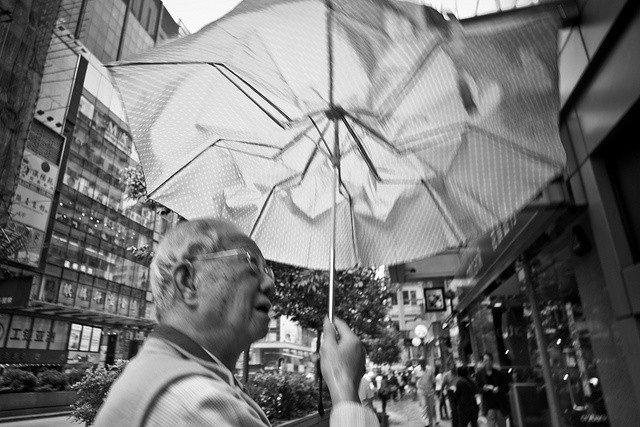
[187,250,274,278]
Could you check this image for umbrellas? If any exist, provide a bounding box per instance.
[101,0,569,326]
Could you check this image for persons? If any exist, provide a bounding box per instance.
[90,218,380,427]
[440,363,459,401]
[411,357,439,427]
[450,365,479,427]
[375,370,409,413]
[475,354,510,427]
[434,364,450,419]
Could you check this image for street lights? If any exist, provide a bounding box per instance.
[411,323,427,362]
